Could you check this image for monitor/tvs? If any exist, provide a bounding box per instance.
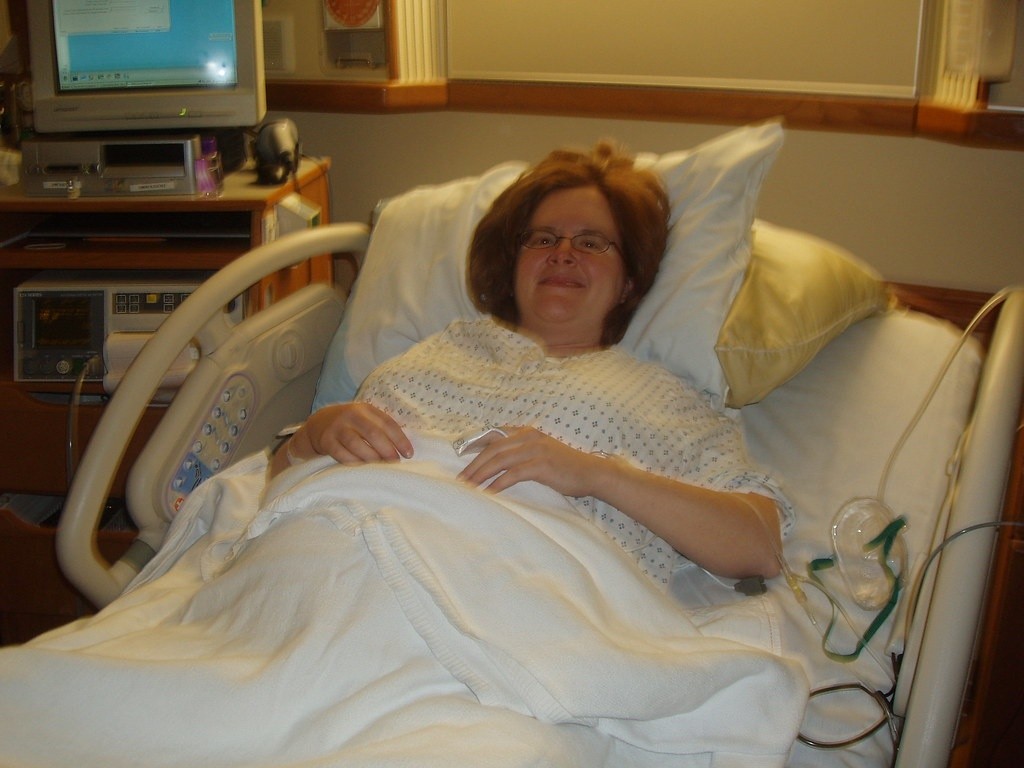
[26,0,268,134]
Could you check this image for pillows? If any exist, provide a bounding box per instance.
[311,116,785,416]
[713,216,888,409]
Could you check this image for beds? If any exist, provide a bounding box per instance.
[0,221,1024,768]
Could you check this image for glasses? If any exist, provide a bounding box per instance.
[515,224,628,263]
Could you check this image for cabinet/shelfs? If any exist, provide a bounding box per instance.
[0,159,331,647]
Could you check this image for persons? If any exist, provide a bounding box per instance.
[264,140,797,594]
